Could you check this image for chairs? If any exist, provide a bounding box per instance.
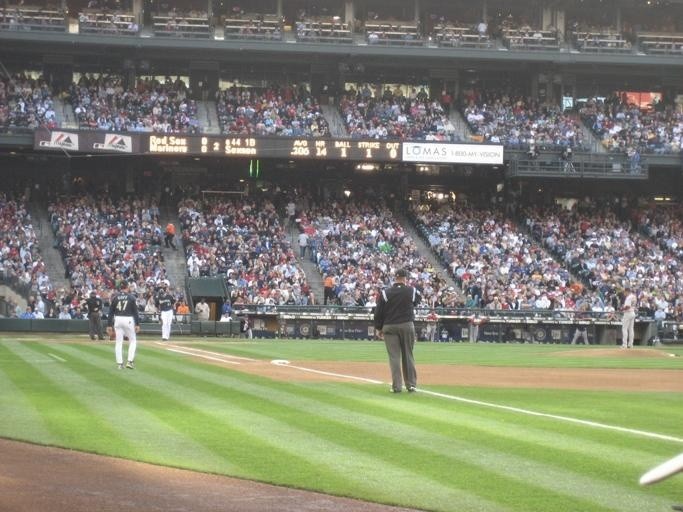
[1,1,682,158]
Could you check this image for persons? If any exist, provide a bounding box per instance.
[373,267,421,392]
[107,281,138,369]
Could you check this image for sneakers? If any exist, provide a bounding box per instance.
[126,361,135,369]
[118,363,126,369]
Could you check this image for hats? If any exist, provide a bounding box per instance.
[393,269,406,278]
[120,281,128,289]
